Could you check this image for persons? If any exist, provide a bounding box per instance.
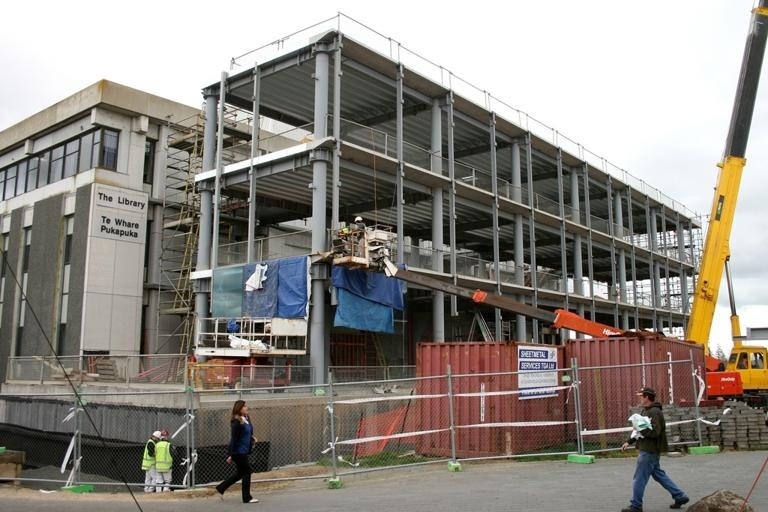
[620,386,690,512]
[141,430,163,492]
[350,216,367,258]
[152,430,174,494]
[214,399,260,503]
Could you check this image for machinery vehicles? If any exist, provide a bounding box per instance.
[328,216,745,411]
[681,1,768,426]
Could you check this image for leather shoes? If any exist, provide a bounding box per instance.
[670,497,689,508]
[621,505,643,511]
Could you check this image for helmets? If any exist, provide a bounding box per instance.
[161,431,169,438]
[153,431,161,438]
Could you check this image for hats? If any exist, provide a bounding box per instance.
[636,387,656,395]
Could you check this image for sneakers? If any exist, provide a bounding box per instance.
[250,499,258,503]
[216,489,224,500]
